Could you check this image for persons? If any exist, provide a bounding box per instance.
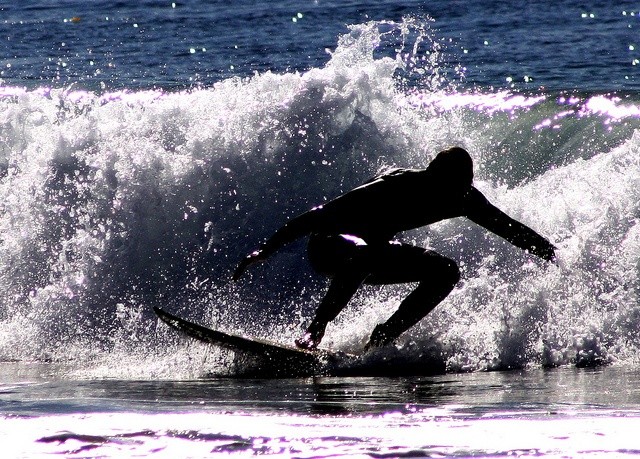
[228,146,562,369]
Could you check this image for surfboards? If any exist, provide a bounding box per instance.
[151,305,361,367]
